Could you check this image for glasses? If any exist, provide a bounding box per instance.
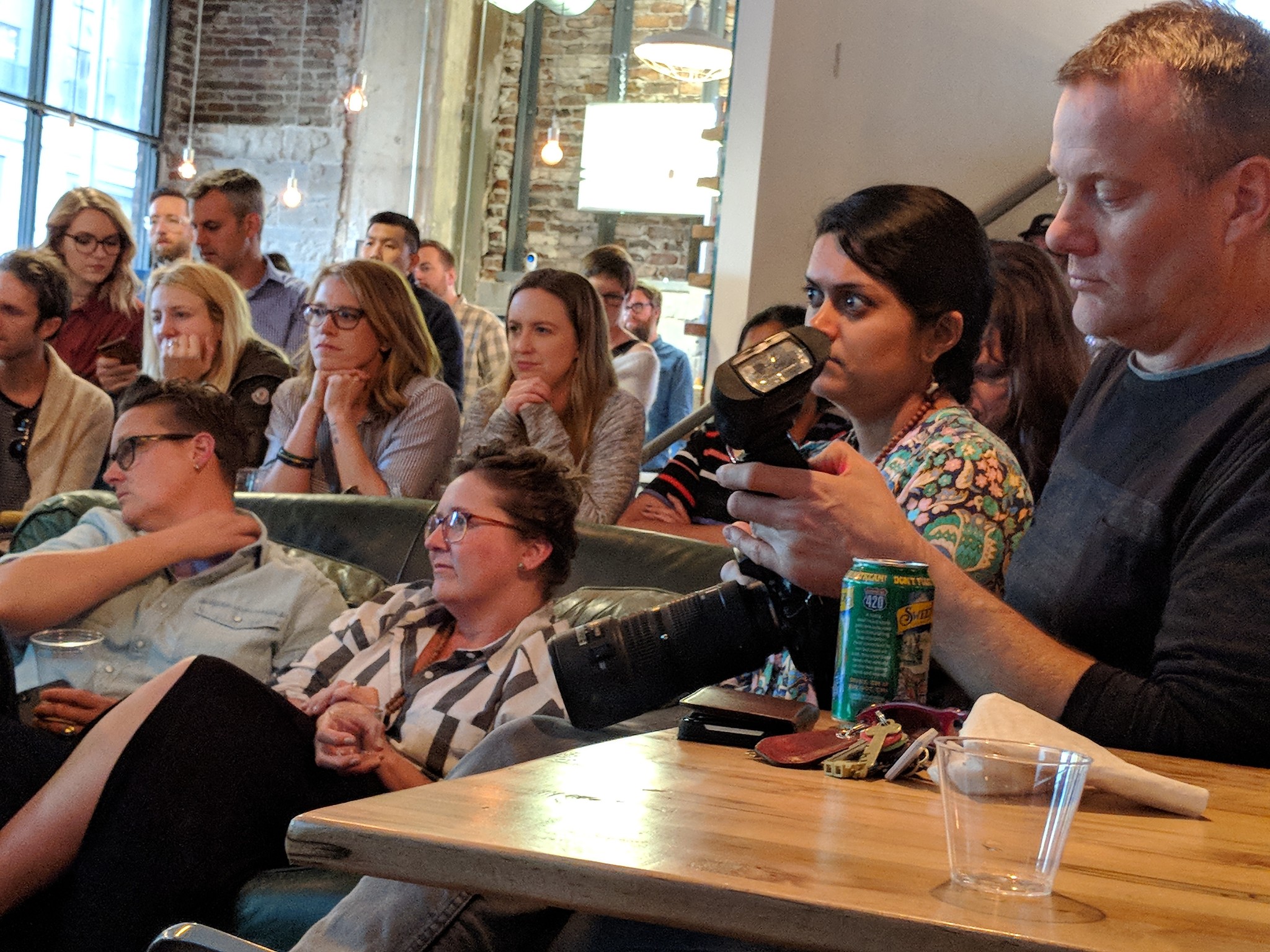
[103,432,194,472]
[601,291,630,308]
[626,302,653,313]
[62,230,122,256]
[425,508,523,544]
[302,305,366,331]
[10,407,32,465]
[144,214,188,232]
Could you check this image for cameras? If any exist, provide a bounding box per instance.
[544,322,858,732]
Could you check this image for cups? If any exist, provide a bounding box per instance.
[235,469,265,493]
[327,703,387,769]
[29,629,105,733]
[934,736,1093,898]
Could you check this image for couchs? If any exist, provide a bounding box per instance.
[0,490,743,952]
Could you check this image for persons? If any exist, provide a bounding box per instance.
[578,245,660,427]
[615,306,852,548]
[362,211,464,412]
[412,239,510,429]
[0,376,350,766]
[289,0,1270,952]
[968,237,1091,500]
[458,268,646,524]
[257,259,461,500]
[1018,214,1057,251]
[0,438,581,952]
[619,282,695,472]
[0,167,306,544]
[731,183,1034,715]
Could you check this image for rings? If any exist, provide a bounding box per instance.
[168,339,178,346]
[352,680,360,688]
[64,725,75,735]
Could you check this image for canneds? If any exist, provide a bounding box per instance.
[830,556,936,724]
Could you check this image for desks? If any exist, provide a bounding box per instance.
[285,709,1270,952]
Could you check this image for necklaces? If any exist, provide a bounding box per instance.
[386,619,453,712]
[873,386,944,465]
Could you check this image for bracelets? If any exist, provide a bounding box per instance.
[277,446,318,469]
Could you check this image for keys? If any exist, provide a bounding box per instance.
[830,716,929,778]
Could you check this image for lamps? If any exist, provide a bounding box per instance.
[634,0,732,83]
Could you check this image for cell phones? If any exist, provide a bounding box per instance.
[98,336,138,367]
[884,728,938,781]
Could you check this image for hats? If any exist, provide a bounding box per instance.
[1019,213,1057,238]
